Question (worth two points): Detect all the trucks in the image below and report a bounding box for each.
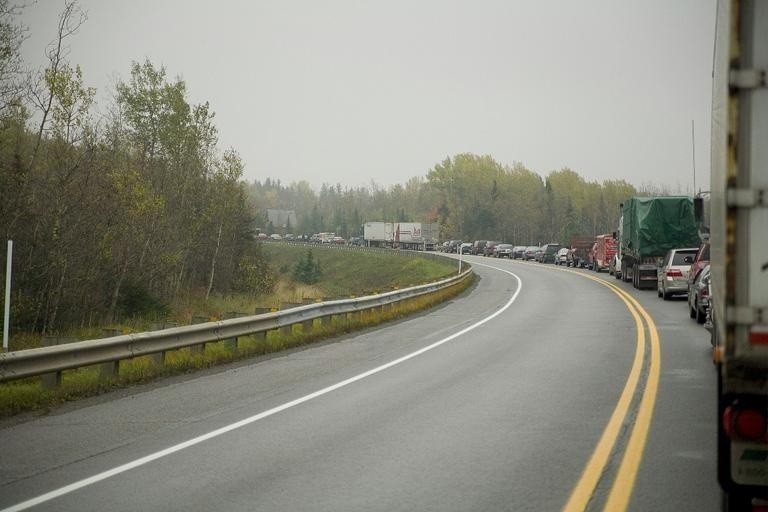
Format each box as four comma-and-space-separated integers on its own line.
693, 0, 768, 510
567, 197, 700, 323
363, 222, 440, 250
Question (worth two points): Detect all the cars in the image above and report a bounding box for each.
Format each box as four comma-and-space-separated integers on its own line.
658, 240, 712, 324
258, 232, 368, 245
442, 240, 567, 264
